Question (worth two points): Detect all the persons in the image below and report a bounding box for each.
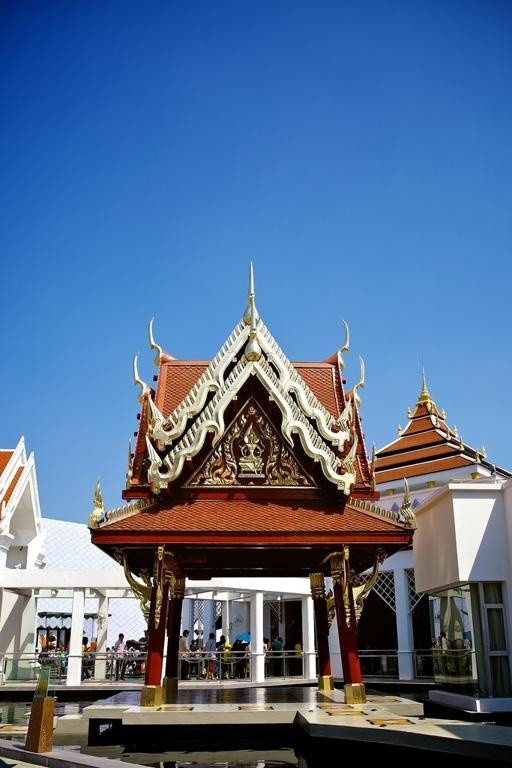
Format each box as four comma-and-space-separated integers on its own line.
81, 630, 303, 681
